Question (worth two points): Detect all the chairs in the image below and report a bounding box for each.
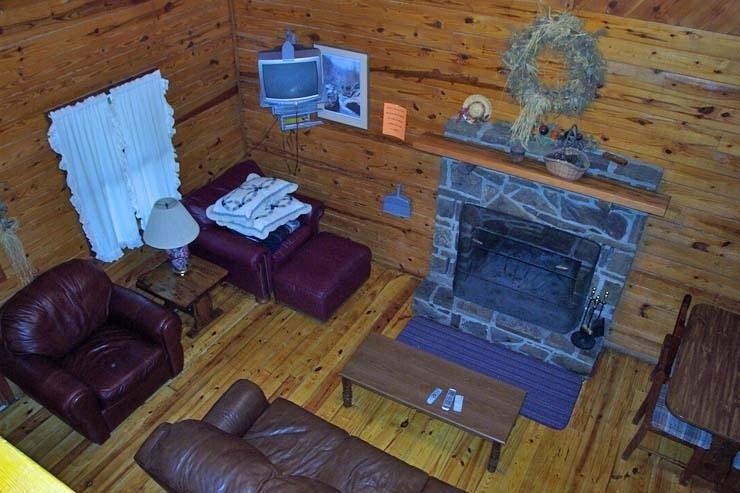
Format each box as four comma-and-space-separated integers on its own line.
674, 293, 693, 332
182, 159, 325, 303
0, 258, 185, 444
621, 336, 711, 485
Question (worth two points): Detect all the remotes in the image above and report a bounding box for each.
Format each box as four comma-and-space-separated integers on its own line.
441, 388, 456, 412
426, 388, 442, 405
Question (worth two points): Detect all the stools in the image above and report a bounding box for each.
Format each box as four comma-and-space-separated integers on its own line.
271, 230, 371, 319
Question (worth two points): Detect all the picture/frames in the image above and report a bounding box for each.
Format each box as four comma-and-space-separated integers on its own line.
313, 42, 369, 130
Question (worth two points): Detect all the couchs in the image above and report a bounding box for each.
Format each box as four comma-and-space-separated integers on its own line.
134, 381, 466, 493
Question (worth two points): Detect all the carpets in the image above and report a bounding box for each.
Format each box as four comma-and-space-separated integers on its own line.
398, 318, 583, 432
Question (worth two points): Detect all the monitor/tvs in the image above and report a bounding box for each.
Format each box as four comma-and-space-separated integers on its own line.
257, 44, 323, 104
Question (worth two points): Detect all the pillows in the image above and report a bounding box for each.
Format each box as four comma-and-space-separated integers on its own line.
207, 173, 297, 223
225, 198, 313, 240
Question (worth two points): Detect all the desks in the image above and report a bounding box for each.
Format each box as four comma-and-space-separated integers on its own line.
136, 255, 228, 336
665, 302, 739, 442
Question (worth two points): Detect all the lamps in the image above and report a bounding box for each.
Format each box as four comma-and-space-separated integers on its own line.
142, 198, 200, 274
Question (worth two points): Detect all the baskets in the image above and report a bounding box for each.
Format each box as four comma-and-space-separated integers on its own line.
544, 124, 591, 180
